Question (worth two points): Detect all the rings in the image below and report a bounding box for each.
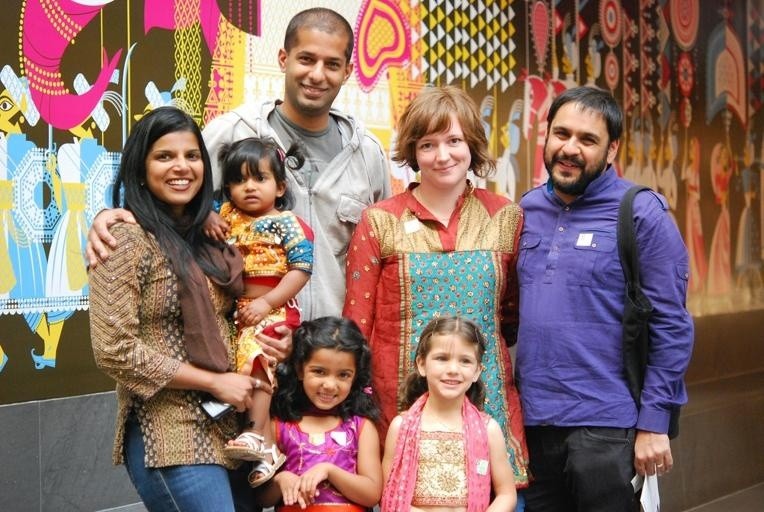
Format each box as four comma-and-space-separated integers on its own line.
253, 378, 262, 390
656, 461, 665, 471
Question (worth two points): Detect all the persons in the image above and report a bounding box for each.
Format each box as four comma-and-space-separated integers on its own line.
265, 315, 383, 512
86, 7, 395, 326
379, 315, 519, 511
86, 106, 278, 510
342, 88, 537, 511
203, 133, 315, 487
509, 86, 695, 512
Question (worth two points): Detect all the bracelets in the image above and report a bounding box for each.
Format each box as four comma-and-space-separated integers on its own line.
257, 293, 276, 313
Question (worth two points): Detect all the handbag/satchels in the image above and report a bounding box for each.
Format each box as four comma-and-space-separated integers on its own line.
623, 282, 679, 439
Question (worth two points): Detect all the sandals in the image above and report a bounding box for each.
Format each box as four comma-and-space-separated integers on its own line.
248, 444, 287, 488
225, 432, 266, 461
